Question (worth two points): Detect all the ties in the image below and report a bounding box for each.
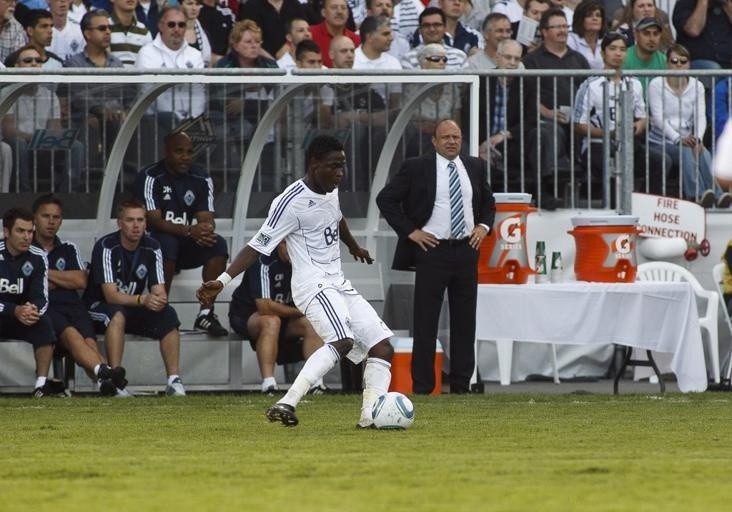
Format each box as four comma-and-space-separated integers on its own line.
447, 162, 465, 240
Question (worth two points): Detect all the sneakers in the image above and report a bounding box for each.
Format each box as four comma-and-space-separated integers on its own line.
101, 364, 128, 390
34, 378, 67, 397
307, 382, 337, 395
354, 421, 378, 430
716, 192, 731, 208
119, 389, 136, 399
100, 378, 117, 397
265, 404, 298, 427
194, 306, 228, 337
261, 381, 279, 393
165, 378, 186, 397
700, 189, 715, 208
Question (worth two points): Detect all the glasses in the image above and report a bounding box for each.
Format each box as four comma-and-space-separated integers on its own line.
421, 22, 444, 29
544, 25, 570, 30
238, 40, 263, 44
497, 51, 522, 62
85, 25, 113, 32
670, 57, 688, 64
163, 21, 186, 28
425, 55, 448, 63
16, 57, 43, 64
487, 27, 514, 35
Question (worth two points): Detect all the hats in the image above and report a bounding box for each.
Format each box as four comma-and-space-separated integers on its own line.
635, 17, 663, 33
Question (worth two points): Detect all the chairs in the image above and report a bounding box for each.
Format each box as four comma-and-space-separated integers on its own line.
630, 260, 722, 388
467, 336, 566, 389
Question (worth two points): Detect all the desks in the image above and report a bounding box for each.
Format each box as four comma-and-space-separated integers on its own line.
391, 279, 695, 397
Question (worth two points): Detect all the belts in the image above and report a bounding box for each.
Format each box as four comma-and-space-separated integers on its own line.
438, 236, 471, 245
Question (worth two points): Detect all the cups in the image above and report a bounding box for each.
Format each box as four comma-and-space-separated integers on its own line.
533, 239, 549, 284
551, 249, 567, 283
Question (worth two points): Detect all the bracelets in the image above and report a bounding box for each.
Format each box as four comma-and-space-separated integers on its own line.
135, 295, 142, 306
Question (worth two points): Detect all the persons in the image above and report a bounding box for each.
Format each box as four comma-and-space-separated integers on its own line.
412, 0, 478, 53
366, 0, 410, 61
227, 238, 344, 399
44, 0, 88, 66
196, 134, 395, 431
0, 207, 67, 399
572, 33, 672, 210
210, 20, 280, 182
134, 6, 211, 165
521, 9, 593, 211
510, 0, 553, 57
714, 117, 732, 189
134, 131, 230, 337
309, 0, 360, 67
461, 39, 536, 167
105, 0, 153, 71
375, 117, 497, 396
4, 8, 64, 70
0, 46, 84, 195
327, 35, 389, 170
349, 15, 403, 109
29, 192, 129, 398
270, 38, 334, 160
647, 44, 732, 210
566, 0, 608, 70
0, 0, 30, 65
400, 6, 468, 91
708, 77, 732, 150
63, 9, 136, 180
672, 0, 732, 89
239, 0, 309, 38
621, 16, 669, 107
275, 19, 314, 67
551, 0, 575, 32
616, 0, 672, 49
460, 12, 525, 73
81, 193, 185, 399
401, 43, 462, 136
178, 0, 212, 68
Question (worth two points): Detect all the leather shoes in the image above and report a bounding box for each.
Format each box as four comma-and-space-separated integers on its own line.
535, 193, 563, 210
451, 389, 470, 394
